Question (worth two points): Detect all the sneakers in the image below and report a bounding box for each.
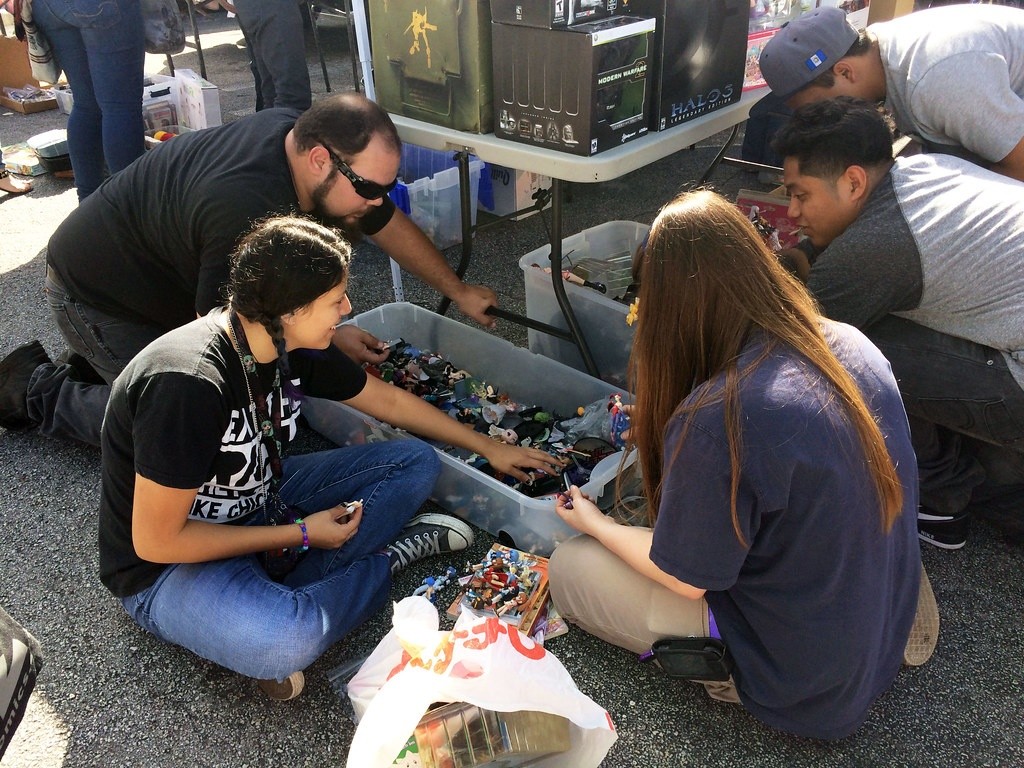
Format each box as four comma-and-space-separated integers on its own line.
256, 671, 305, 701
378, 511, 473, 575
912, 500, 968, 551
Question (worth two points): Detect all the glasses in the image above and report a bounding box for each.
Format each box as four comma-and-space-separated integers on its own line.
302, 132, 398, 200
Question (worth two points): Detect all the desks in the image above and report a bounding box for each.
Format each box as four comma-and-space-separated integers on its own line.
385, 85, 787, 380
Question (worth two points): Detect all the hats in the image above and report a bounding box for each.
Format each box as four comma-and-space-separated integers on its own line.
748, 8, 860, 119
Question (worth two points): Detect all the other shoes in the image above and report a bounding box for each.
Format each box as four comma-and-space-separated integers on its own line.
0, 340, 48, 433
897, 558, 939, 665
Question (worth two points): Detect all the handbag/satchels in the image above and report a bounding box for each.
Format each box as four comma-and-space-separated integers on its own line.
139, 0, 185, 53
346, 594, 618, 768
19, 0, 64, 84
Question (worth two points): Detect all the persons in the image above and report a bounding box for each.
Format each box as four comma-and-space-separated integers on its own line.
98, 210, 567, 702
770, 95, 1024, 551
546, 189, 940, 743
0, 149, 33, 193
0, 0, 312, 206
0, 92, 499, 450
742, 90, 792, 175
358, 339, 622, 620
759, 3, 1024, 184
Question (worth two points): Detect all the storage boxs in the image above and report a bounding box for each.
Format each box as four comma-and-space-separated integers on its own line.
300, 302, 639, 557
518, 220, 652, 391
477, 160, 551, 221
387, 140, 485, 251
0, 34, 222, 175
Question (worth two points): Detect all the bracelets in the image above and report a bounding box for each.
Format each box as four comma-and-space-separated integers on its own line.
291, 517, 308, 553
